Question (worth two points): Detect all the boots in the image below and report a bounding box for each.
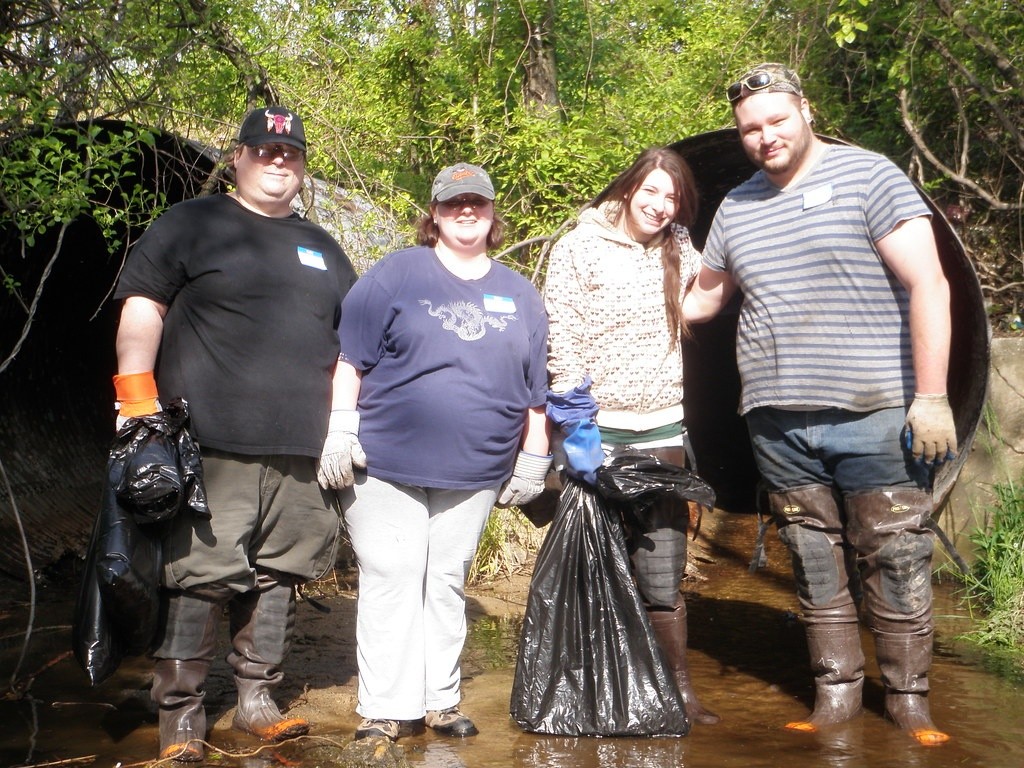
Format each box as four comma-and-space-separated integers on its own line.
767, 482, 866, 731
627, 446, 721, 726
226, 570, 310, 742
150, 582, 229, 762
845, 487, 951, 747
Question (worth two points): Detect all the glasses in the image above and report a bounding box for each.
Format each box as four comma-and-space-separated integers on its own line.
726, 73, 799, 102
253, 143, 300, 160
443, 197, 489, 211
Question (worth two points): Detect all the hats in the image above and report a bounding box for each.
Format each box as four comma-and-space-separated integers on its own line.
239, 107, 306, 151
734, 63, 802, 104
431, 162, 495, 203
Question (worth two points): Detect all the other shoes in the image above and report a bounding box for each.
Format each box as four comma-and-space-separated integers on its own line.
355, 718, 399, 742
424, 706, 478, 738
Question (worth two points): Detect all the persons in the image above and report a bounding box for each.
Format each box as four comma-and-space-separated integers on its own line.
543, 146, 738, 721
682, 63, 959, 744
316, 163, 553, 741
112, 107, 363, 762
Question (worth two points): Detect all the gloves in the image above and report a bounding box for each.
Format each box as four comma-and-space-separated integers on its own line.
112, 371, 163, 434
905, 393, 957, 466
315, 411, 368, 490
546, 377, 604, 485
498, 450, 553, 505
